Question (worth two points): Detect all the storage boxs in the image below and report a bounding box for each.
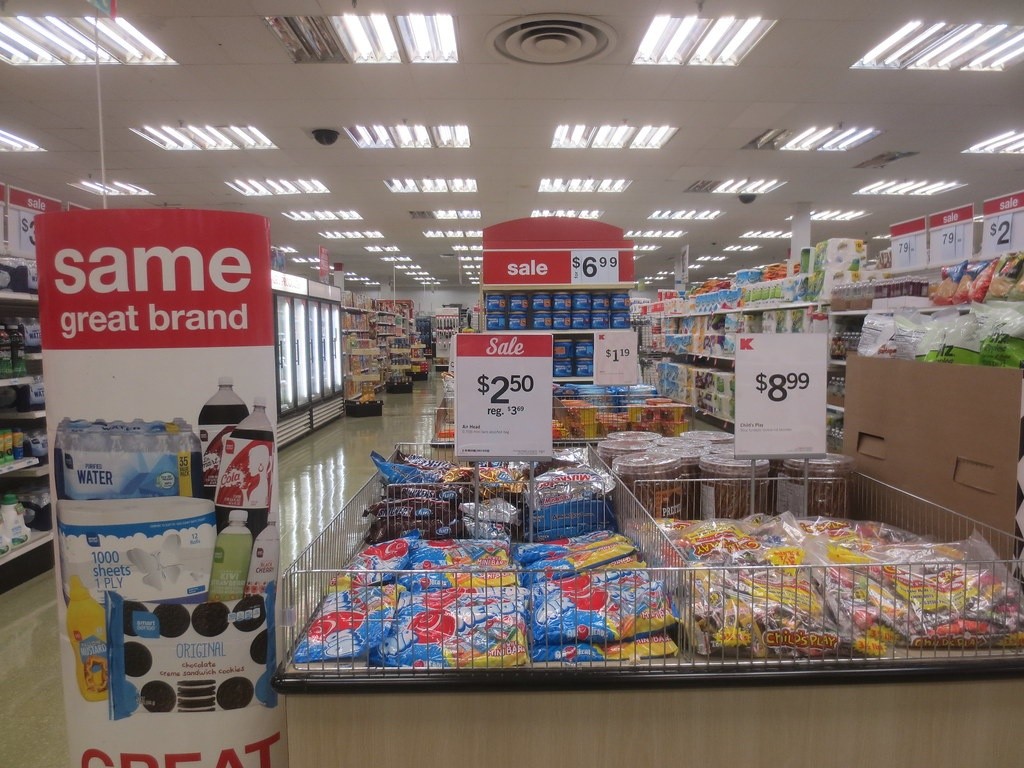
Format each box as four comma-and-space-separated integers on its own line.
406, 371, 428, 381
436, 365, 449, 371
386, 380, 413, 394
346, 402, 383, 417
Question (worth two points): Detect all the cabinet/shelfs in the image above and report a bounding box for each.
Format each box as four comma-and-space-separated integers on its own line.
628, 257, 998, 427
0, 290, 58, 593
340, 306, 425, 400
480, 283, 635, 382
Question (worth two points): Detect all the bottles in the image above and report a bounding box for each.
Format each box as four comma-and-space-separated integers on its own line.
214, 396, 275, 537
242, 512, 280, 601
2, 494, 31, 549
53, 417, 205, 499
0, 316, 41, 376
206, 510, 253, 605
199, 374, 250, 503
0, 428, 24, 463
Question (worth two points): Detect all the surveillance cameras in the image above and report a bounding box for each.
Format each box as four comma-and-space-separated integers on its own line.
738, 194, 757, 204
311, 130, 340, 146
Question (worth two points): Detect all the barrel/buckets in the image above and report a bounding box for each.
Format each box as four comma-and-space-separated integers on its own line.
598, 430, 857, 521
